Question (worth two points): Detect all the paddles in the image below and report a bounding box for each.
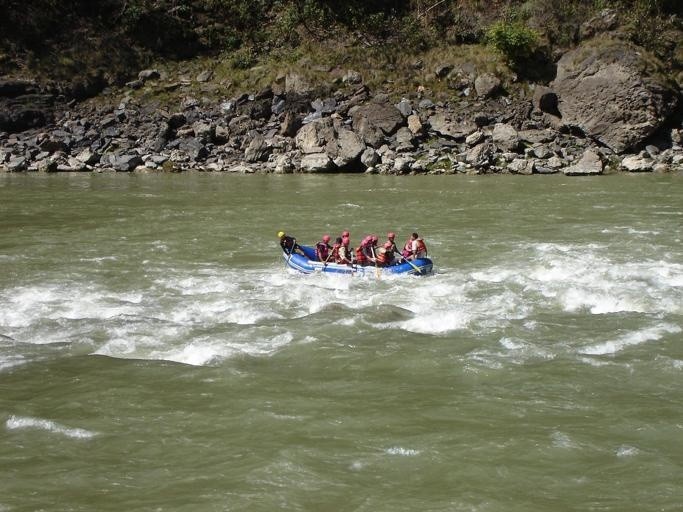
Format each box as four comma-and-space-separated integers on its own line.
394, 248, 422, 274
370, 246, 381, 278
351, 248, 358, 277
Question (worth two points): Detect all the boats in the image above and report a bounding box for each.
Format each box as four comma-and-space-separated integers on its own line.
282, 243, 434, 279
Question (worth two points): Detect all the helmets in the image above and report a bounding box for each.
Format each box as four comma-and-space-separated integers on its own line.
322, 231, 350, 245
278, 231, 285, 238
366, 233, 395, 241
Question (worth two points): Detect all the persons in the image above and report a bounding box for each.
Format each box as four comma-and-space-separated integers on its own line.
314, 231, 427, 268
277, 231, 311, 260
